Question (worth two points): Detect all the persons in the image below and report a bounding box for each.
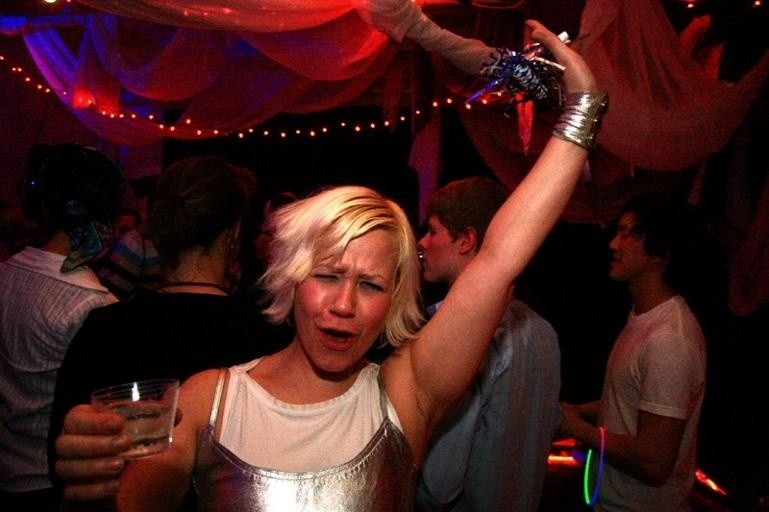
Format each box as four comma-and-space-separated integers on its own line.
54, 20, 608, 512
556, 193, 708, 512
1, 142, 297, 512
413, 175, 562, 512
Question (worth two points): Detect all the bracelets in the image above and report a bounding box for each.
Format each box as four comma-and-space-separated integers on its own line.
551, 92, 609, 152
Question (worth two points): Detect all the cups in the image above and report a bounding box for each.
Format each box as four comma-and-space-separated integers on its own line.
89, 377, 180, 461
